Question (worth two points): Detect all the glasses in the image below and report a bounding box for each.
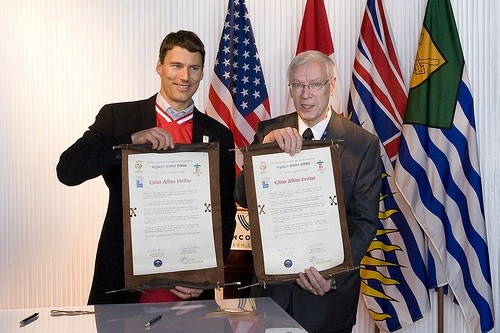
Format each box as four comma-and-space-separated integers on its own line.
287, 78, 331, 91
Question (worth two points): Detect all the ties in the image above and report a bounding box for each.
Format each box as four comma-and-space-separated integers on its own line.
302, 129, 317, 142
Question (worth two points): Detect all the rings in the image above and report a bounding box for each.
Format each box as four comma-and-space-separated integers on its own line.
308, 286, 314, 292
188, 293, 192, 299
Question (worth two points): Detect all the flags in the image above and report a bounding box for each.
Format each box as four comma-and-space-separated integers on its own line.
395, 0, 495, 333
208, 0, 271, 179
295, 0, 335, 57
347, 0, 458, 332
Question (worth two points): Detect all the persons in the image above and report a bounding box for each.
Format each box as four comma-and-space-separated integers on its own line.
233, 50, 384, 333
56, 30, 236, 305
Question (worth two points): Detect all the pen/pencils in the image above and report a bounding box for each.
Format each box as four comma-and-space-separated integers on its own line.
145, 315, 162, 326
20, 313, 39, 324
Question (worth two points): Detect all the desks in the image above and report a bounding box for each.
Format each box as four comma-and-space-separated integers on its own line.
1, 297, 308, 333
